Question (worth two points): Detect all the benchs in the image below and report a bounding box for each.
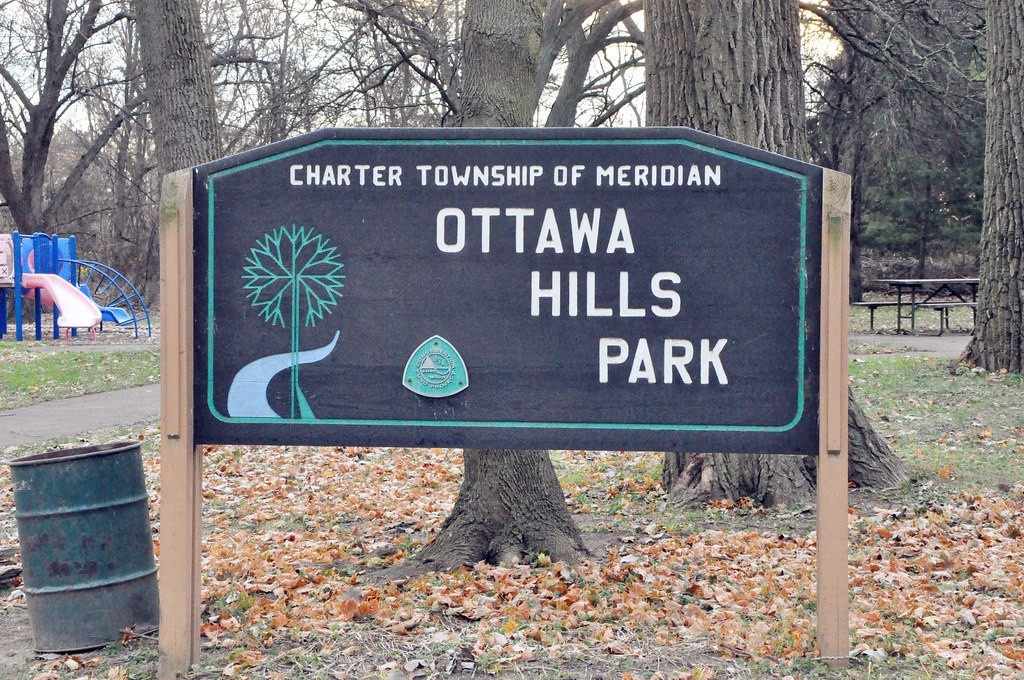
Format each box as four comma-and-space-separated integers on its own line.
851, 299, 978, 335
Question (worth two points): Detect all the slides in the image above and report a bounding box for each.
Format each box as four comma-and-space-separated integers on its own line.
20, 273, 103, 327
76, 281, 131, 324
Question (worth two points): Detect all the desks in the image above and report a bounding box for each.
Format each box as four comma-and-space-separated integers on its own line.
872, 278, 985, 336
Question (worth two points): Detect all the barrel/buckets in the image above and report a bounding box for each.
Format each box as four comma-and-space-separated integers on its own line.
7, 440, 160, 653
